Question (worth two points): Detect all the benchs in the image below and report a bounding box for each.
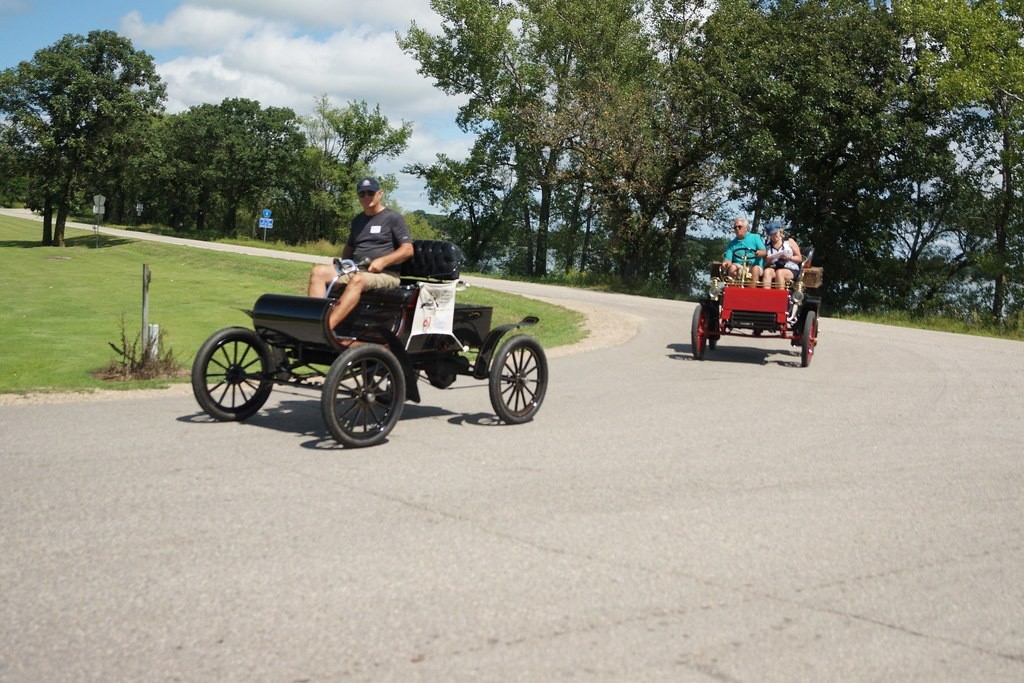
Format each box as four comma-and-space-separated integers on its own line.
325, 238, 462, 336
794, 246, 816, 281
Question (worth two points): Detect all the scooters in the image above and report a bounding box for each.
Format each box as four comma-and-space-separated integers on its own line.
690, 246, 823, 367
191, 240, 550, 448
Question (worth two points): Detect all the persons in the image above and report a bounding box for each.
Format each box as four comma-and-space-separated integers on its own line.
718, 217, 767, 289
308, 177, 415, 331
762, 222, 803, 290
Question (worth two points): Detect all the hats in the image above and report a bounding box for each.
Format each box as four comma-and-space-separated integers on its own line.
357, 178, 380, 193
765, 222, 781, 234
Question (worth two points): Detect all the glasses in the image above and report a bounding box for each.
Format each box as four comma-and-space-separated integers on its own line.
734, 225, 746, 230
357, 191, 379, 198
